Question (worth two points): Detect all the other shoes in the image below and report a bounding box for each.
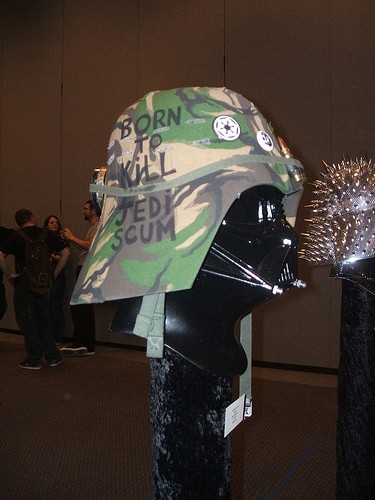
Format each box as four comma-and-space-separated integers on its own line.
18, 358, 42, 370
55, 342, 72, 351
59, 344, 95, 356
48, 352, 64, 366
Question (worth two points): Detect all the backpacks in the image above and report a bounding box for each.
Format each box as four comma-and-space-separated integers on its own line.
16, 228, 52, 295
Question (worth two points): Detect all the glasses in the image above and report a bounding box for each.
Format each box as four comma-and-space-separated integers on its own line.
48, 221, 58, 224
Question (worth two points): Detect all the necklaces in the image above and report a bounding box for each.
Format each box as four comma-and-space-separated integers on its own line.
90, 218, 99, 226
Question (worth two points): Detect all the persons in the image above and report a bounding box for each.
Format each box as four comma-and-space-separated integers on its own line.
57, 200, 103, 356
0, 199, 70, 369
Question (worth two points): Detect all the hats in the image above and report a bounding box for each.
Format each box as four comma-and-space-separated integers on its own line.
69, 83, 306, 306
